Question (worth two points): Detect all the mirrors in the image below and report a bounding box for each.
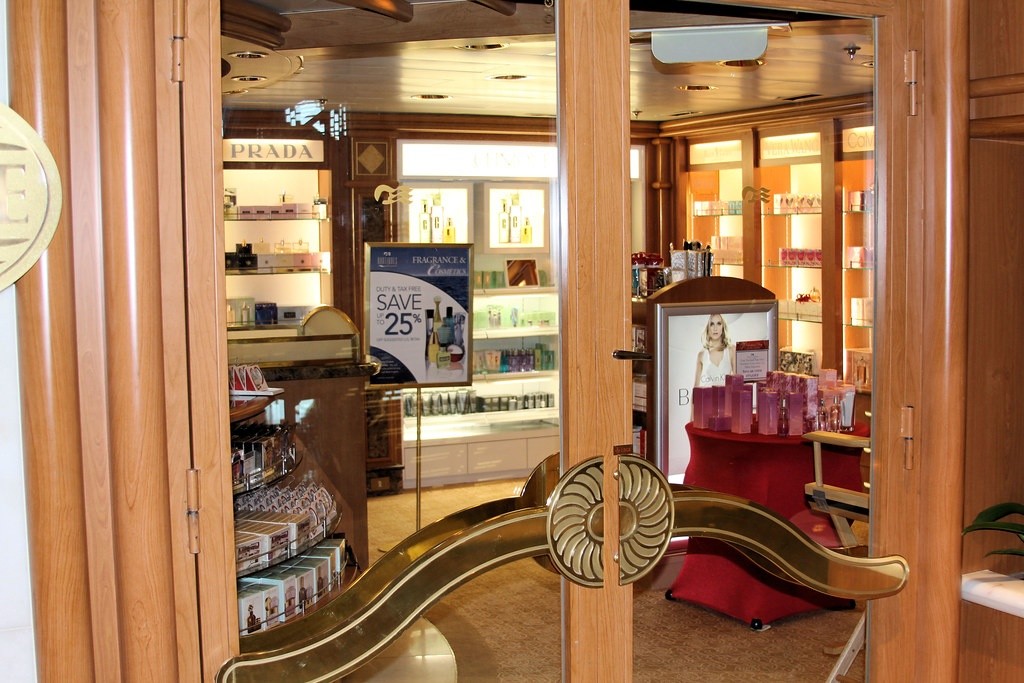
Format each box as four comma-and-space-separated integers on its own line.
504, 258, 540, 289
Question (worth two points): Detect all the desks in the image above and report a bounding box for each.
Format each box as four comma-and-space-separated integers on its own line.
666, 419, 871, 635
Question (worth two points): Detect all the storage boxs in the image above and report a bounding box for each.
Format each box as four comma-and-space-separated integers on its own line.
690, 345, 872, 435
850, 296, 874, 328
848, 190, 864, 211
227, 364, 349, 636
710, 236, 743, 265
692, 199, 742, 216
226, 242, 330, 272
475, 310, 555, 328
473, 269, 547, 290
226, 297, 313, 326
778, 247, 823, 268
847, 245, 874, 268
777, 298, 822, 317
473, 343, 556, 375
773, 193, 822, 213
238, 202, 312, 220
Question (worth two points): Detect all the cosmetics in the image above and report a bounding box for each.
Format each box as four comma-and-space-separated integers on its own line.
773, 191, 873, 386
230, 399, 345, 636
224, 193, 742, 458
692, 370, 855, 436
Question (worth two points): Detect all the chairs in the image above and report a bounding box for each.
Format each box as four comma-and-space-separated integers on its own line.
800, 425, 876, 683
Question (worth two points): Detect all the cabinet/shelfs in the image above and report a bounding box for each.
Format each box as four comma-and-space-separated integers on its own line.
226, 396, 359, 639
396, 138, 648, 491
671, 110, 878, 429
220, 162, 336, 333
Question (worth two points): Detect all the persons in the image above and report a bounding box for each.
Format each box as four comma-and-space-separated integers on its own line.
695, 313, 738, 386
